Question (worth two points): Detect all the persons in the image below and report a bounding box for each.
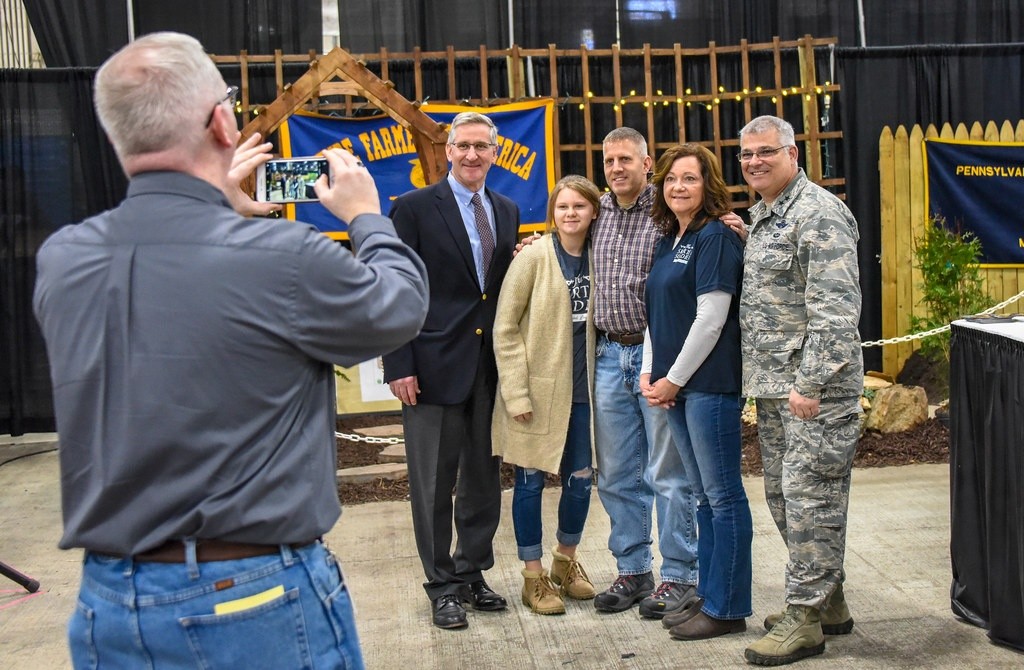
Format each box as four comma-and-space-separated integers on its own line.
512, 128, 747, 617
281, 173, 304, 199
382, 113, 522, 630
640, 144, 754, 640
491, 177, 599, 613
739, 117, 863, 665
33, 33, 428, 670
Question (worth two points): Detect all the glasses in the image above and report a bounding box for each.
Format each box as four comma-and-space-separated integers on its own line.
205, 86, 240, 129
449, 140, 496, 151
737, 144, 792, 163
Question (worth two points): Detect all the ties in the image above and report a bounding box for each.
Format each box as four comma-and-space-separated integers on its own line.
471, 193, 495, 293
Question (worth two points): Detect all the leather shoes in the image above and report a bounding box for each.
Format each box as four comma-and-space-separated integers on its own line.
431, 596, 466, 628
669, 601, 748, 641
454, 579, 507, 611
662, 592, 705, 630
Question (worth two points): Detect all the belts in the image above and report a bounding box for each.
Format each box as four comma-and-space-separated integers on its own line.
92, 533, 315, 562
598, 328, 644, 347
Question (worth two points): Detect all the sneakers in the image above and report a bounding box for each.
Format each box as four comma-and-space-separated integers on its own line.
551, 545, 595, 600
521, 566, 566, 614
594, 566, 655, 612
640, 578, 701, 618
762, 587, 854, 634
744, 600, 824, 664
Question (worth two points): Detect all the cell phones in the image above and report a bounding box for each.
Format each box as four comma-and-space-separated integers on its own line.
254, 156, 334, 204
